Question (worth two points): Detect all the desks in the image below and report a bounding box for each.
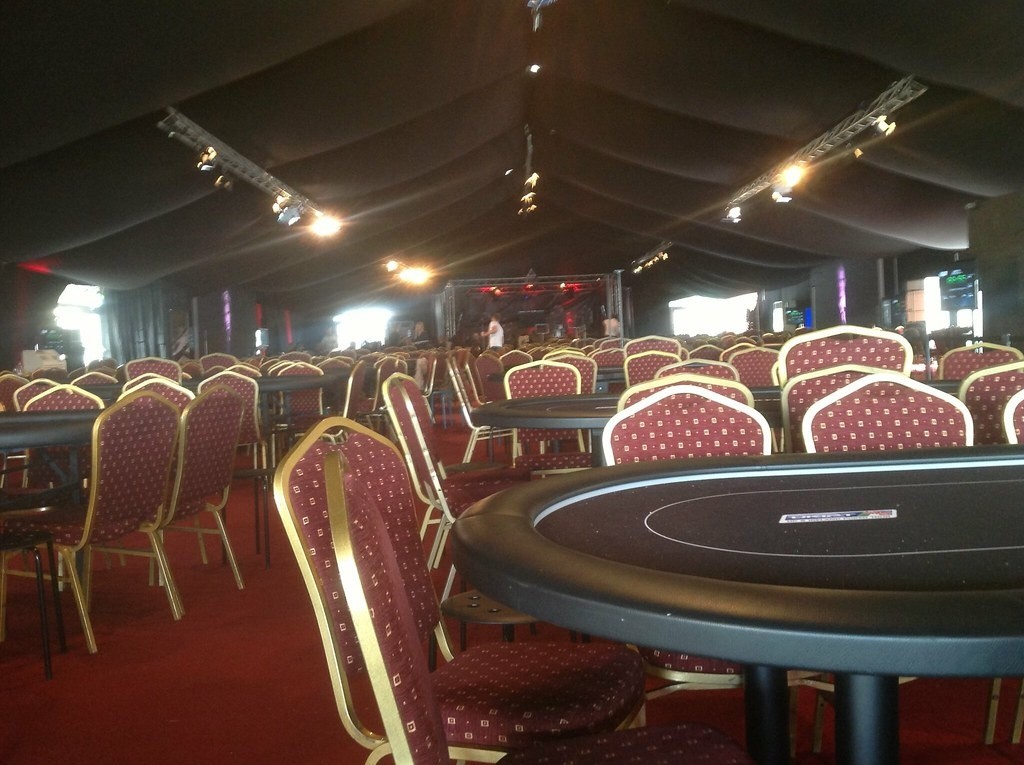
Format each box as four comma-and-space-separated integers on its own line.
485, 363, 627, 394
448, 443, 1024, 765
88, 374, 335, 437
469, 378, 962, 469
0, 408, 108, 589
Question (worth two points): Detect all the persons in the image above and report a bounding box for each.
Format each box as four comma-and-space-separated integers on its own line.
482, 313, 504, 348
602, 314, 621, 337
321, 321, 429, 352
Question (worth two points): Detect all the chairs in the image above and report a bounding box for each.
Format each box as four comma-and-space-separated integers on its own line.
0, 323, 1024, 765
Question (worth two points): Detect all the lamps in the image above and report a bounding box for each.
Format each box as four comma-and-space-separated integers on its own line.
871, 116, 892, 133
720, 202, 743, 224
270, 194, 302, 228
628, 236, 674, 277
189, 143, 232, 193
771, 169, 794, 202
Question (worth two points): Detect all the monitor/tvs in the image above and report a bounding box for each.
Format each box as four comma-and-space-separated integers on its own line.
940, 273, 979, 311
